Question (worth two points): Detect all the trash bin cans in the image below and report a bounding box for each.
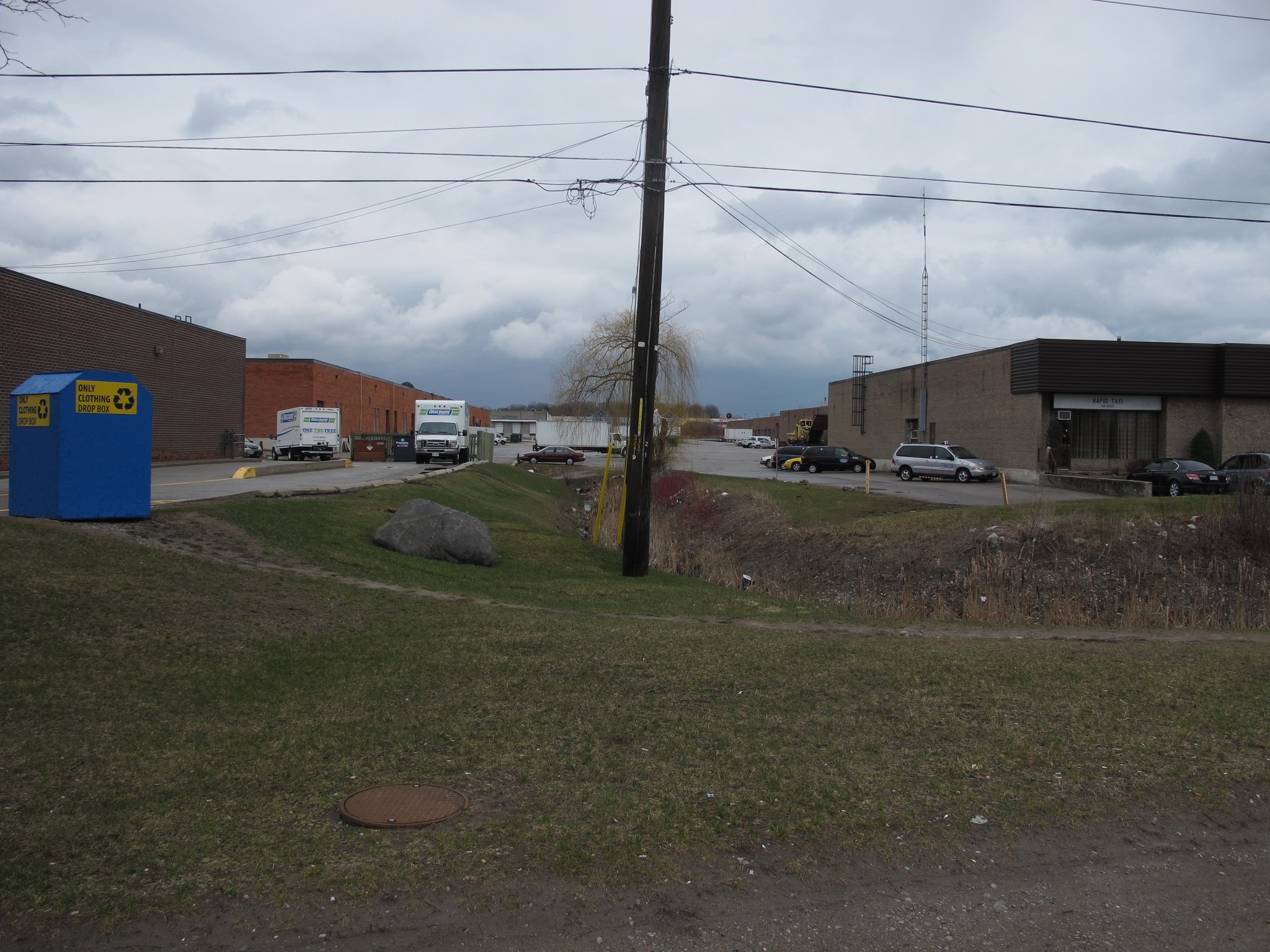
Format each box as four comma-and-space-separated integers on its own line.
393, 435, 415, 462
510, 434, 520, 442
354, 439, 386, 461
9, 369, 154, 519
512, 433, 523, 442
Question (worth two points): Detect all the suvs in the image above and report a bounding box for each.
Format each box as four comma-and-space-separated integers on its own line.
892, 441, 999, 483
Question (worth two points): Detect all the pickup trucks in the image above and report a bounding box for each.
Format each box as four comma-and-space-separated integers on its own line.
742, 437, 771, 448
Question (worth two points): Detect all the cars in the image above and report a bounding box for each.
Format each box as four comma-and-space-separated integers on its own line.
751, 440, 776, 449
522, 447, 586, 465
735, 439, 745, 446
784, 457, 802, 472
1129, 458, 1230, 497
771, 446, 808, 470
1218, 451, 1270, 496
760, 455, 779, 468
494, 437, 500, 445
801, 446, 876, 473
244, 437, 263, 459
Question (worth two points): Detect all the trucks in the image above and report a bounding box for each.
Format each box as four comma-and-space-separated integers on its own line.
494, 433, 508, 445
269, 407, 340, 461
535, 419, 626, 456
410, 399, 470, 465
